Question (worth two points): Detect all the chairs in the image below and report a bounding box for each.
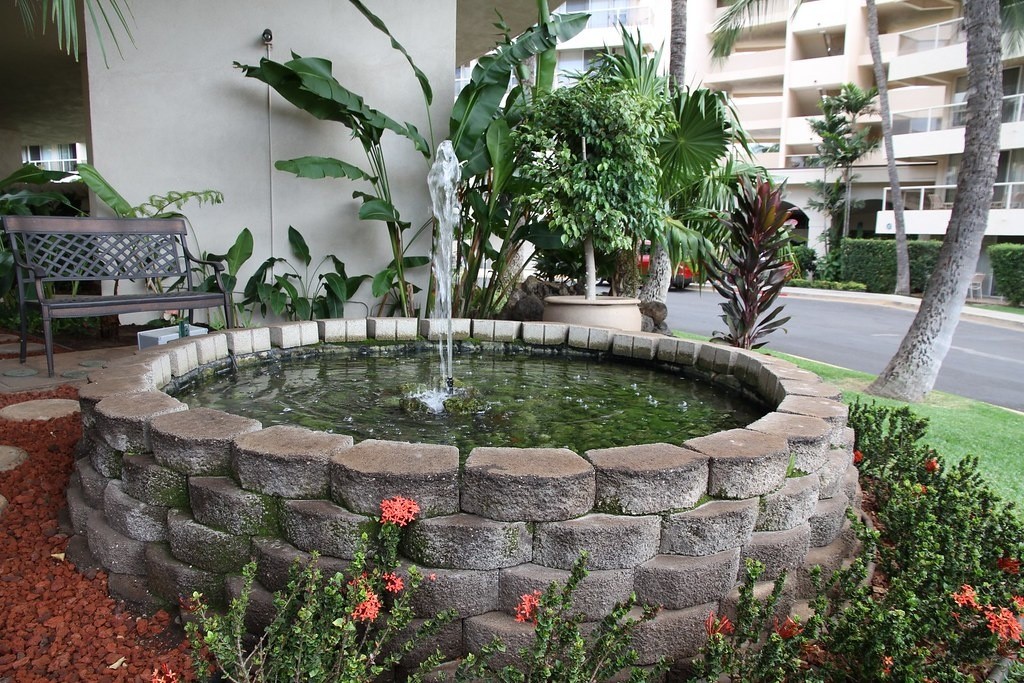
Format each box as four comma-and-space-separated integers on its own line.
969, 273, 985, 298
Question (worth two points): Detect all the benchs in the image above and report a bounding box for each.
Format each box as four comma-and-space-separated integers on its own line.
2, 215, 234, 379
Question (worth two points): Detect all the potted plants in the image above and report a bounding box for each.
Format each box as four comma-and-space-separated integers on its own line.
509, 76, 681, 332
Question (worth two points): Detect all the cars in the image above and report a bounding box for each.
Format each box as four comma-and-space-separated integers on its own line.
616, 240, 708, 290
